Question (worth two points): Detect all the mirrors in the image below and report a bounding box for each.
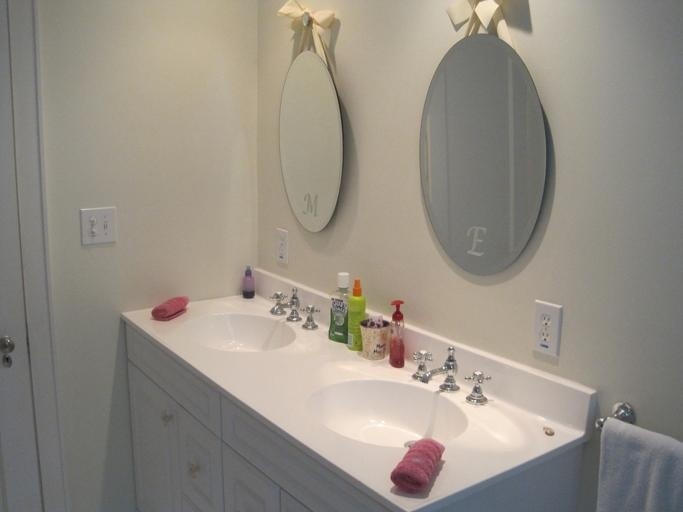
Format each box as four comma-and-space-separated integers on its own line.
419, 32, 547, 276
278, 50, 345, 234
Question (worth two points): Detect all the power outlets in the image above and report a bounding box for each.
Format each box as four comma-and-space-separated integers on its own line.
532, 299, 563, 357
275, 228, 289, 265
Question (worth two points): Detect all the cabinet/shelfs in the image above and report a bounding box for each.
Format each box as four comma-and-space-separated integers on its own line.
124, 322, 223, 511
218, 395, 394, 512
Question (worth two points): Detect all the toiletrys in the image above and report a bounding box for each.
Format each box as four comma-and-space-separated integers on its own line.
328, 272, 351, 343
389, 300, 405, 368
242, 264, 255, 298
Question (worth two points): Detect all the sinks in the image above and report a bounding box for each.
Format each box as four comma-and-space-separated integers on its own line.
309, 379, 468, 448
181, 314, 296, 352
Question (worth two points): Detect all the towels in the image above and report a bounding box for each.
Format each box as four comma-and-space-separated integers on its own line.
390, 438, 446, 492
151, 295, 189, 322
596, 417, 683, 512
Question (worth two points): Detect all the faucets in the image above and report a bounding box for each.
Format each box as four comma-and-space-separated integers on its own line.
271, 287, 302, 322
420, 346, 459, 392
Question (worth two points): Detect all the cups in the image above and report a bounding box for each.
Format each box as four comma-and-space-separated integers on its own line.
359, 319, 390, 360
